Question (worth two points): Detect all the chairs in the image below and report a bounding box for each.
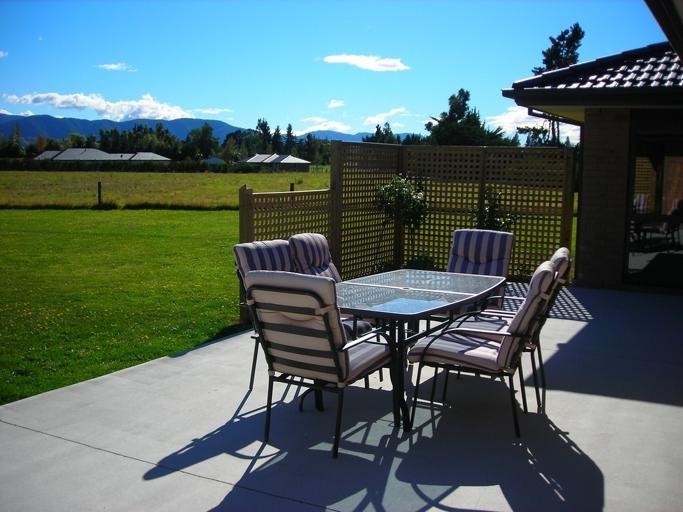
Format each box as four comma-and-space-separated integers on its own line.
244, 270, 401, 458
226, 238, 295, 430
453, 247, 574, 416
629, 193, 683, 263
289, 233, 376, 337
425, 230, 516, 337
408, 260, 558, 442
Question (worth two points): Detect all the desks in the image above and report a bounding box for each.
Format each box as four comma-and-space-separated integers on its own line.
332, 267, 507, 423
